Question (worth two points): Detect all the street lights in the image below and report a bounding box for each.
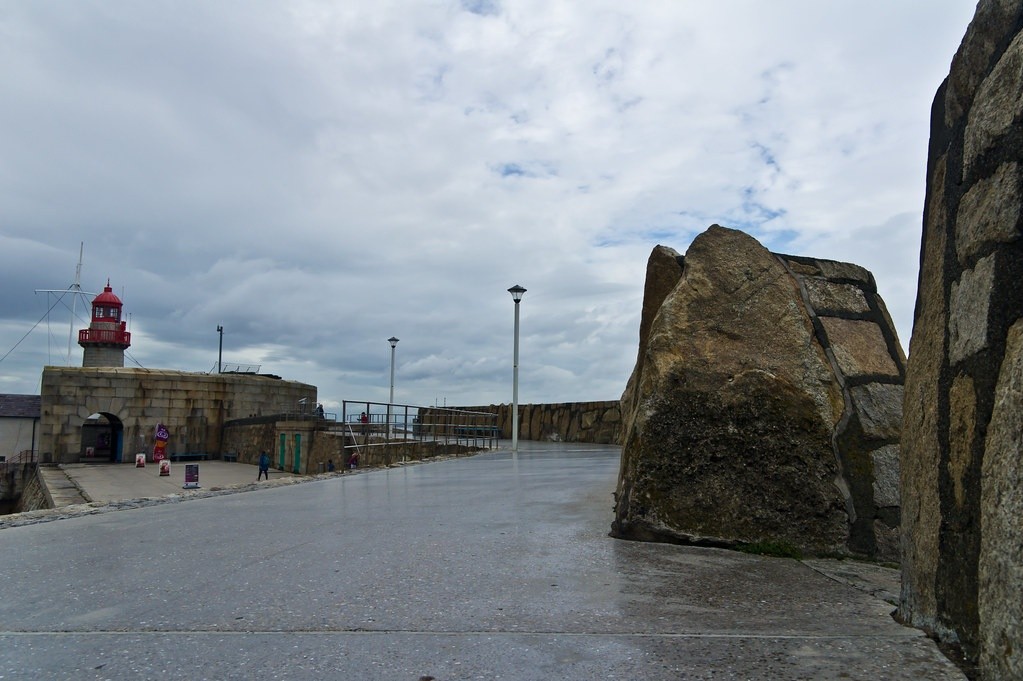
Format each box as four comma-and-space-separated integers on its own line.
508, 285, 527, 452
387, 336, 400, 439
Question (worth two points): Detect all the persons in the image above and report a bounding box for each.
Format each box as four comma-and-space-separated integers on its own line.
315, 404, 324, 419
256, 451, 270, 481
327, 458, 335, 472
356, 412, 368, 435
346, 453, 357, 469
